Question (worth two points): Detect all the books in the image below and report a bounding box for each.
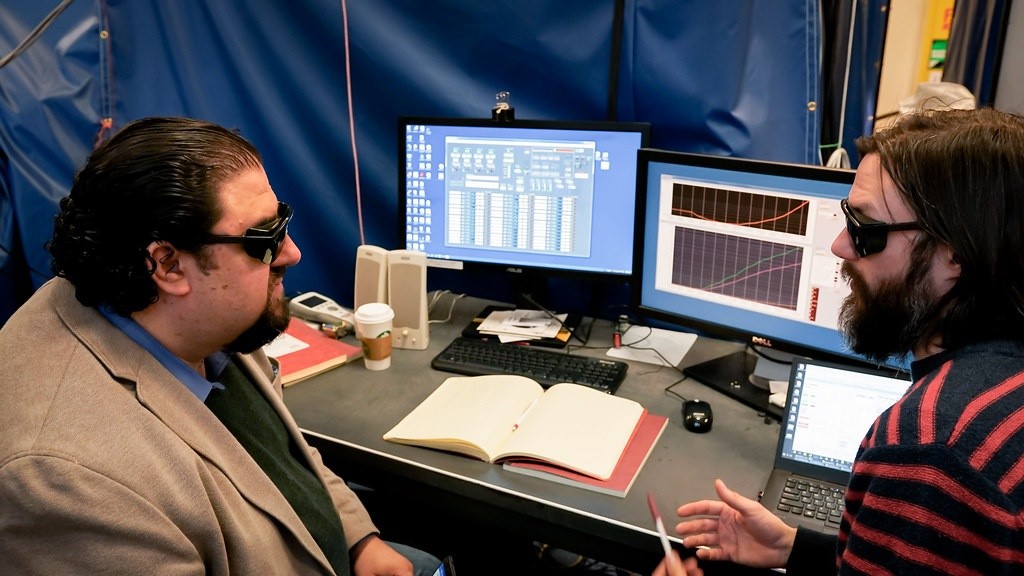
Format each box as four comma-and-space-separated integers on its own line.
380, 373, 672, 498
261, 313, 367, 388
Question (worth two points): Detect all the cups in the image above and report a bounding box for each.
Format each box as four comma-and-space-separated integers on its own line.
354, 303, 395, 372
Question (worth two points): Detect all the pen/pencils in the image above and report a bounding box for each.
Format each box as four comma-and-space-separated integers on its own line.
646, 488, 675, 563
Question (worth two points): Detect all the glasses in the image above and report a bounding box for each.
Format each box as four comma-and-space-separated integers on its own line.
199, 201, 295, 265
840, 197, 925, 258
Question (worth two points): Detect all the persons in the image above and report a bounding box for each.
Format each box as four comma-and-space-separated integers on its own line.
655, 97, 1024, 576
0, 118, 443, 576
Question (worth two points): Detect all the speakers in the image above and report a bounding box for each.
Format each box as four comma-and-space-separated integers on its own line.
354, 245, 389, 310
387, 250, 430, 351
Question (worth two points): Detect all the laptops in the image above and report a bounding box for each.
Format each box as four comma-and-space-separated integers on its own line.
760, 356, 914, 534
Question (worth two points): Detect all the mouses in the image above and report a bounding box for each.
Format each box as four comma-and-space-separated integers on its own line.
681, 398, 714, 433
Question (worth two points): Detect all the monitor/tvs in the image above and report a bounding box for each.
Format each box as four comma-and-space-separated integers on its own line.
397, 116, 652, 349
630, 146, 920, 421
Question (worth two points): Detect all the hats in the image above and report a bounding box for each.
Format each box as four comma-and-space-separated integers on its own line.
896, 81, 977, 115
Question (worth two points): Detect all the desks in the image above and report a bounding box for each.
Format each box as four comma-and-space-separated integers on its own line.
283, 290, 812, 576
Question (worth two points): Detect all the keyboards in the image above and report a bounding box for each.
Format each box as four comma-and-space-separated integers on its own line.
432, 337, 630, 395
